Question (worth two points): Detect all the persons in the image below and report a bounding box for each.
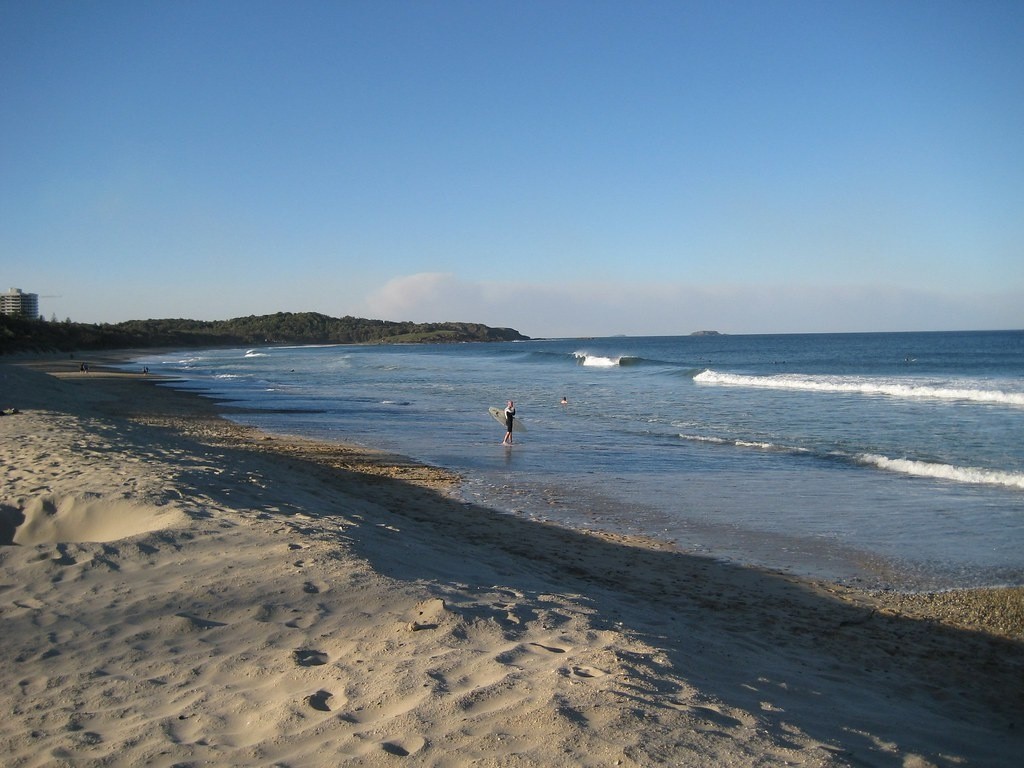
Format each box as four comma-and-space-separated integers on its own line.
561, 397, 568, 404
502, 400, 516, 446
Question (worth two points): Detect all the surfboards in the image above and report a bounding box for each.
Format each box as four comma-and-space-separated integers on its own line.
488, 406, 528, 433
